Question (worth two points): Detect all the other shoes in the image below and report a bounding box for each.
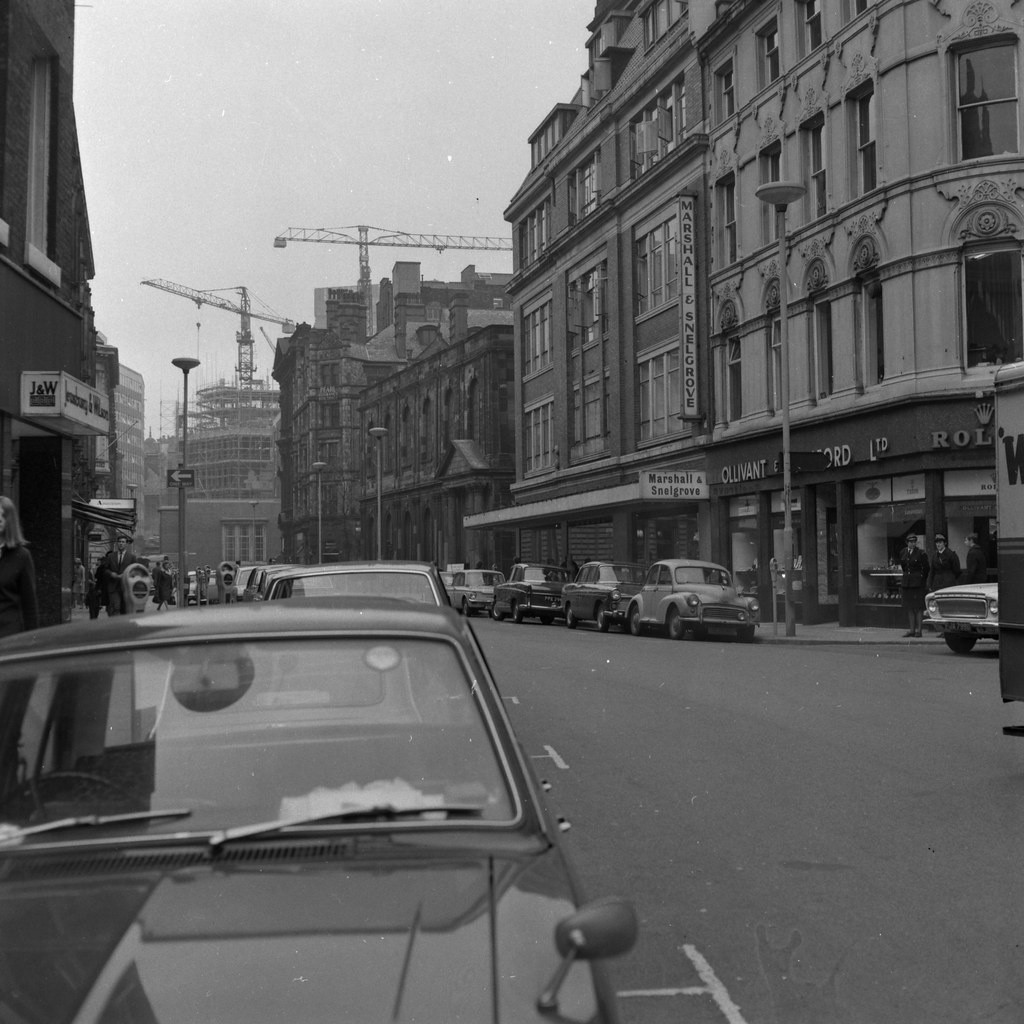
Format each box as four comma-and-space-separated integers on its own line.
916, 632, 922, 638
936, 632, 946, 638
902, 631, 914, 637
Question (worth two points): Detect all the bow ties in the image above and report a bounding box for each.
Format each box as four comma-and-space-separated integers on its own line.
937, 552, 942, 557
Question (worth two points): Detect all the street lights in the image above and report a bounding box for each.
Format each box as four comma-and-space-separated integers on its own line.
369, 426, 389, 560
126, 483, 138, 556
171, 357, 201, 610
756, 182, 810, 638
312, 460, 326, 567
249, 499, 259, 567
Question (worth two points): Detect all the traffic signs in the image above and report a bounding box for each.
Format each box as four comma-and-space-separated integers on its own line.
165, 469, 196, 488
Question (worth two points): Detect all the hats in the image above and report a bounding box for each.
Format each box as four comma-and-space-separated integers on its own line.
934, 534, 945, 542
905, 534, 917, 543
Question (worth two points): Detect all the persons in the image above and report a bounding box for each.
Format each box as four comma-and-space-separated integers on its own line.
71, 536, 279, 622
898, 534, 930, 638
925, 534, 961, 593
430, 553, 593, 593
1, 495, 40, 639
963, 533, 989, 585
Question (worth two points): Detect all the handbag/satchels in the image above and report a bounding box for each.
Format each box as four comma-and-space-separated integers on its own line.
152, 596, 161, 603
169, 596, 176, 605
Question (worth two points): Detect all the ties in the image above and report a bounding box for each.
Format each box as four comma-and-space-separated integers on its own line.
118, 552, 124, 568
908, 549, 911, 559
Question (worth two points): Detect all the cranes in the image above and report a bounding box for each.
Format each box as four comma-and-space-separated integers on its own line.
259, 325, 279, 355
141, 278, 299, 389
272, 222, 514, 340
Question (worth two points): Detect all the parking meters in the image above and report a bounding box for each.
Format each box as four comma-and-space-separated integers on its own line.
215, 560, 238, 605
769, 557, 779, 638
121, 564, 153, 617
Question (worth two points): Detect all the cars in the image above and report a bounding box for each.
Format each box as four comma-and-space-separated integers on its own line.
187, 559, 454, 609
561, 560, 653, 633
491, 563, 574, 625
437, 569, 456, 588
922, 583, 998, 655
445, 569, 507, 617
0, 593, 641, 1023
624, 559, 761, 643
